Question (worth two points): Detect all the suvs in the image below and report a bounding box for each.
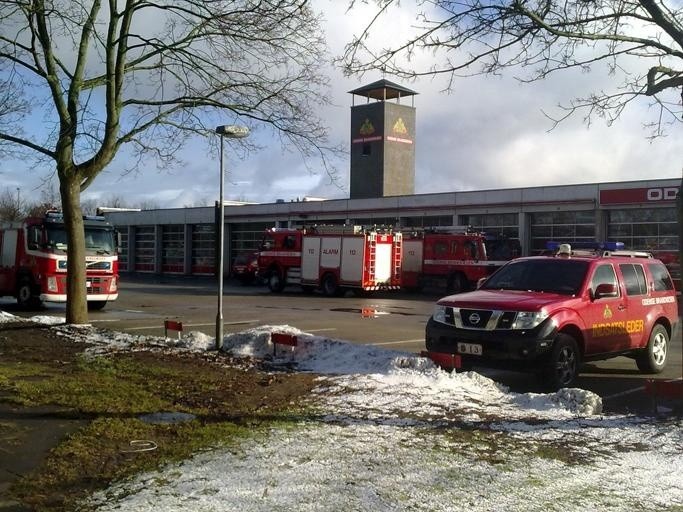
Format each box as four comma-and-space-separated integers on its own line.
230, 251, 269, 287
416, 240, 677, 389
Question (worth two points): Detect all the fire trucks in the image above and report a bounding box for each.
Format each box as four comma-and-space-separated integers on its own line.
398, 225, 522, 293
239, 220, 404, 297
0, 206, 120, 314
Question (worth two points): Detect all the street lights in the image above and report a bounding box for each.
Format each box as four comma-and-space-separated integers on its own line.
214, 122, 251, 351
